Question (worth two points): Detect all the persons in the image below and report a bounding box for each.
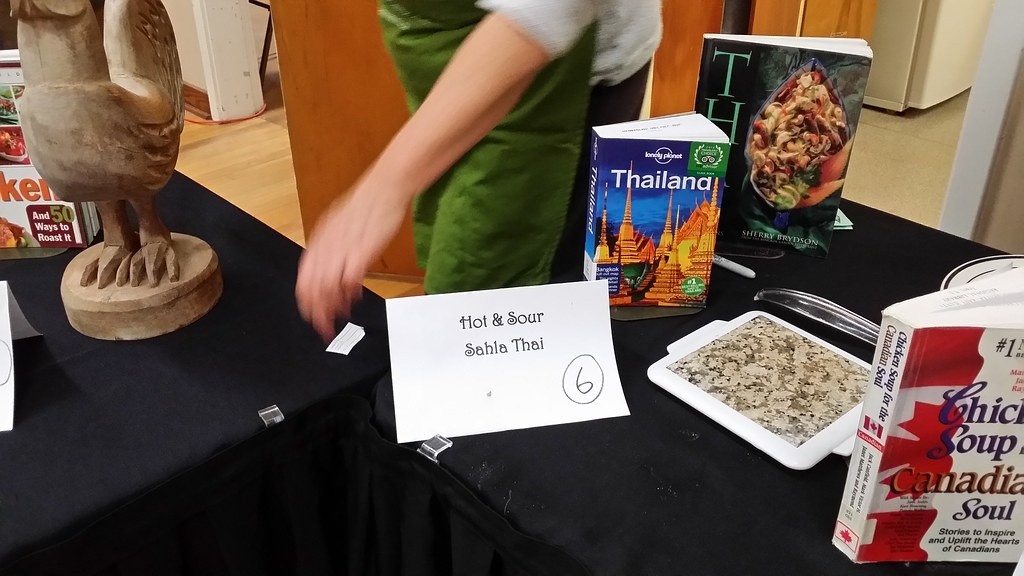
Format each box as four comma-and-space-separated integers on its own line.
293, 0, 664, 343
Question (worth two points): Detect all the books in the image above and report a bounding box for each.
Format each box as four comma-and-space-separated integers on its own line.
582, 111, 731, 309
0, 49, 100, 249
694, 33, 873, 258
831, 265, 1024, 562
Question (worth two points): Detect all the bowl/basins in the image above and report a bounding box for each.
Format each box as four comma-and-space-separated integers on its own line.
0, 149, 27, 162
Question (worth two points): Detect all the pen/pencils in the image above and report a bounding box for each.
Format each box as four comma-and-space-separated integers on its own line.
713, 254, 757, 279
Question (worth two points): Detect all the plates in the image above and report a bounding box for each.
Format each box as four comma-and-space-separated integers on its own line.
940, 255, 1024, 291
13, 87, 23, 95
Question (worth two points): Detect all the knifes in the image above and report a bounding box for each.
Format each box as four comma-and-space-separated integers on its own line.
714, 242, 785, 258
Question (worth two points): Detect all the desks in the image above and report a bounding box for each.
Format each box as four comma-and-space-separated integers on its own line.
0, 171, 401, 576
373, 194, 1022, 575
